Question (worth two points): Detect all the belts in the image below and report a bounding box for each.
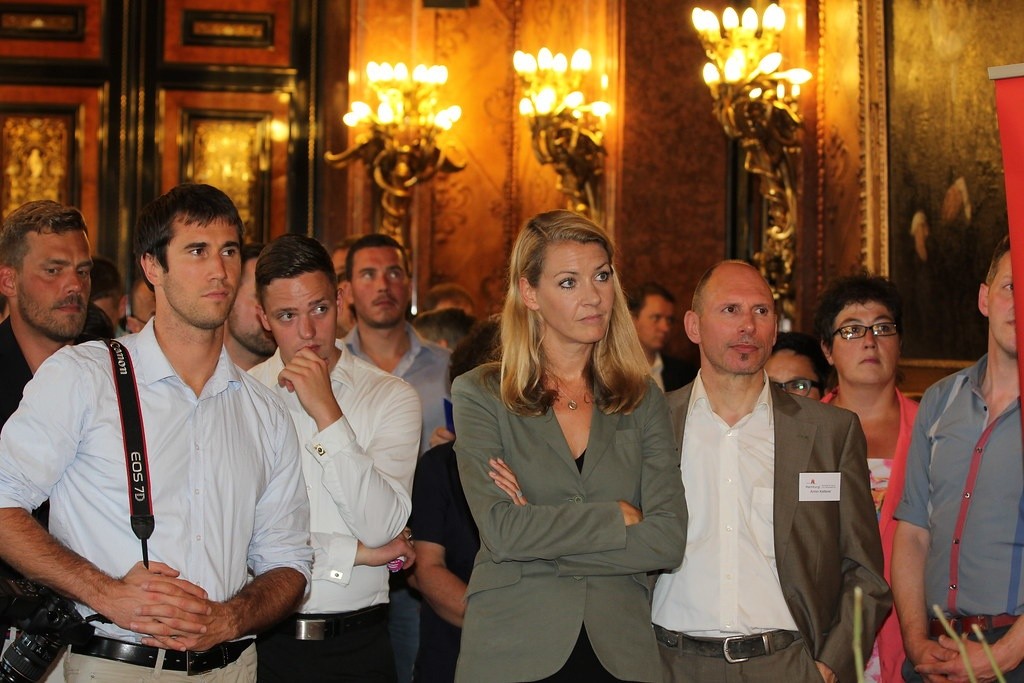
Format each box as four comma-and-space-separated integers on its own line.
274, 603, 386, 643
70, 633, 254, 677
649, 622, 802, 664
928, 613, 1021, 638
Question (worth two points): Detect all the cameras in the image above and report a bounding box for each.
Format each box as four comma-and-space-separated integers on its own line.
0, 569, 95, 683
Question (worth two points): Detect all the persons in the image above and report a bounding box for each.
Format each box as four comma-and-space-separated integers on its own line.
329, 245, 508, 456
224, 243, 279, 372
0, 198, 93, 683
765, 332, 832, 402
74, 255, 156, 346
628, 281, 698, 392
0, 184, 315, 683
814, 275, 920, 683
451, 209, 689, 683
404, 312, 505, 683
888, 232, 1024, 683
342, 234, 455, 683
245, 233, 423, 683
651, 260, 892, 683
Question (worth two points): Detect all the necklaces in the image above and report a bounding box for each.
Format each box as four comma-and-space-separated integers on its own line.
547, 373, 584, 410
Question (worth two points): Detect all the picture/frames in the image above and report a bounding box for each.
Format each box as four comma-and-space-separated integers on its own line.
856, 0, 1024, 369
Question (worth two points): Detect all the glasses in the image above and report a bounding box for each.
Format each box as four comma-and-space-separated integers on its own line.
771, 378, 824, 397
831, 321, 900, 340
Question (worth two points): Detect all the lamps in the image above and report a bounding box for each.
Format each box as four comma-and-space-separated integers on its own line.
513, 47, 613, 226
693, 2, 812, 318
324, 61, 469, 275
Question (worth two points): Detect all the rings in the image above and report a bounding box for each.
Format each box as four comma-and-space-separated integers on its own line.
408, 534, 413, 540
515, 490, 522, 498
